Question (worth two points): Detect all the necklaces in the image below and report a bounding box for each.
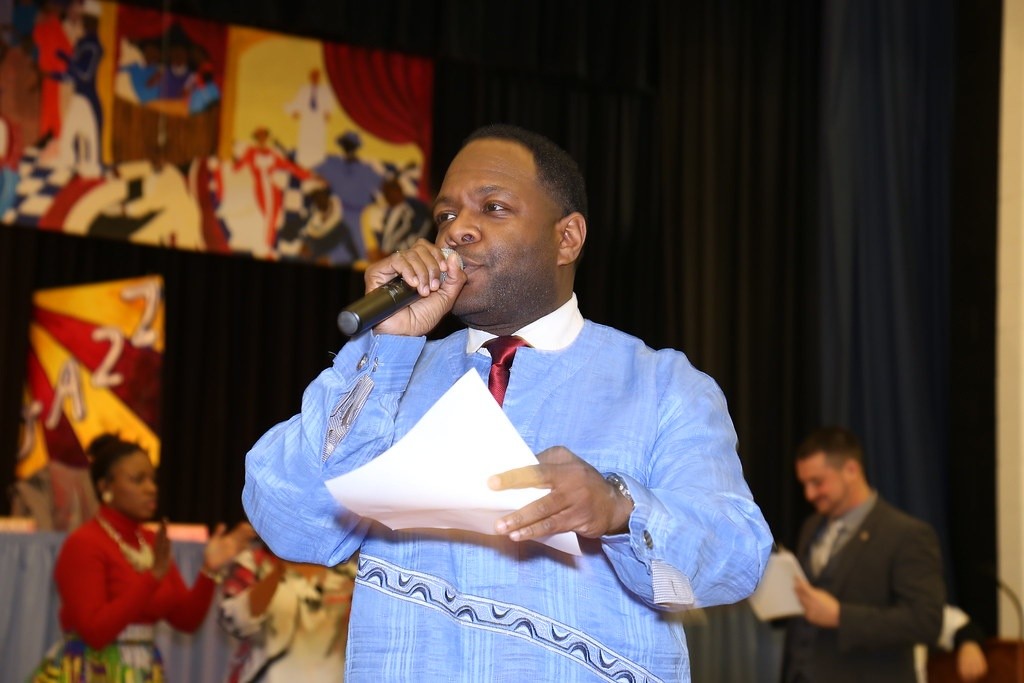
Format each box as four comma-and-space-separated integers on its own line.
96, 505, 156, 571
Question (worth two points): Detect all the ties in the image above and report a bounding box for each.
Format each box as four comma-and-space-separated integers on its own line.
810, 521, 844, 580
481, 336, 535, 407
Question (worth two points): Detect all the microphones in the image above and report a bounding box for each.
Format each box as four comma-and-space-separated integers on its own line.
338, 249, 463, 337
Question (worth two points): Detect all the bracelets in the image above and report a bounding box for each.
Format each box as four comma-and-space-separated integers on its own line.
605, 473, 634, 511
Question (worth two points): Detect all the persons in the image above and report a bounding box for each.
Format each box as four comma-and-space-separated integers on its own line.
744, 423, 950, 683
240, 128, 774, 683
54, 430, 252, 683
218, 532, 360, 683
